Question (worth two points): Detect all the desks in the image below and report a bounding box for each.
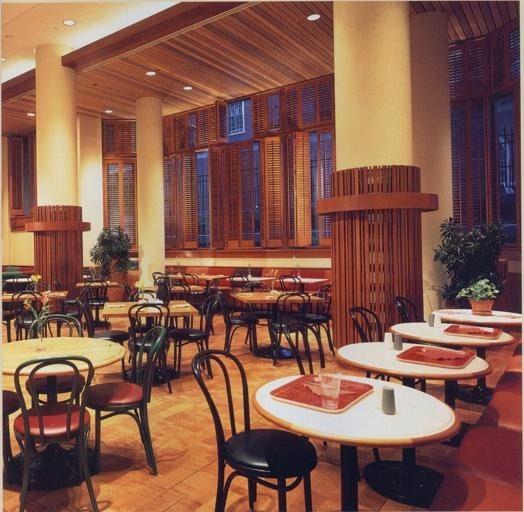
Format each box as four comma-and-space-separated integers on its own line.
335, 308, 524, 379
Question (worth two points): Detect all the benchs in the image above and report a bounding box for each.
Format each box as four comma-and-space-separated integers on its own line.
128, 266, 334, 318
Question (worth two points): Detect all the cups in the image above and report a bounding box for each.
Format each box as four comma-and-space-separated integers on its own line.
320, 368, 341, 408
433, 311, 442, 328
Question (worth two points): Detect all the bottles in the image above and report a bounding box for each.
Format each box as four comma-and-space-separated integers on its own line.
384, 333, 392, 349
382, 387, 396, 415
393, 333, 403, 350
428, 314, 435, 328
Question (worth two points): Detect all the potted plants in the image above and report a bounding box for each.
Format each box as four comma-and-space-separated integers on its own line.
88, 224, 134, 302
456, 279, 500, 316
